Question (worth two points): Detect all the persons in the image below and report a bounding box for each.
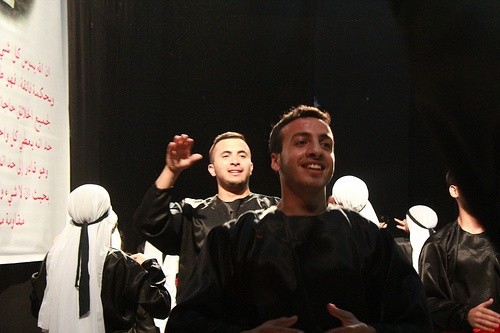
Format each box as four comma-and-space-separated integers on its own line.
417, 168, 500, 332
134, 130, 280, 305
30, 184, 171, 333
163, 105, 434, 333
331, 175, 396, 247
394, 205, 438, 277
